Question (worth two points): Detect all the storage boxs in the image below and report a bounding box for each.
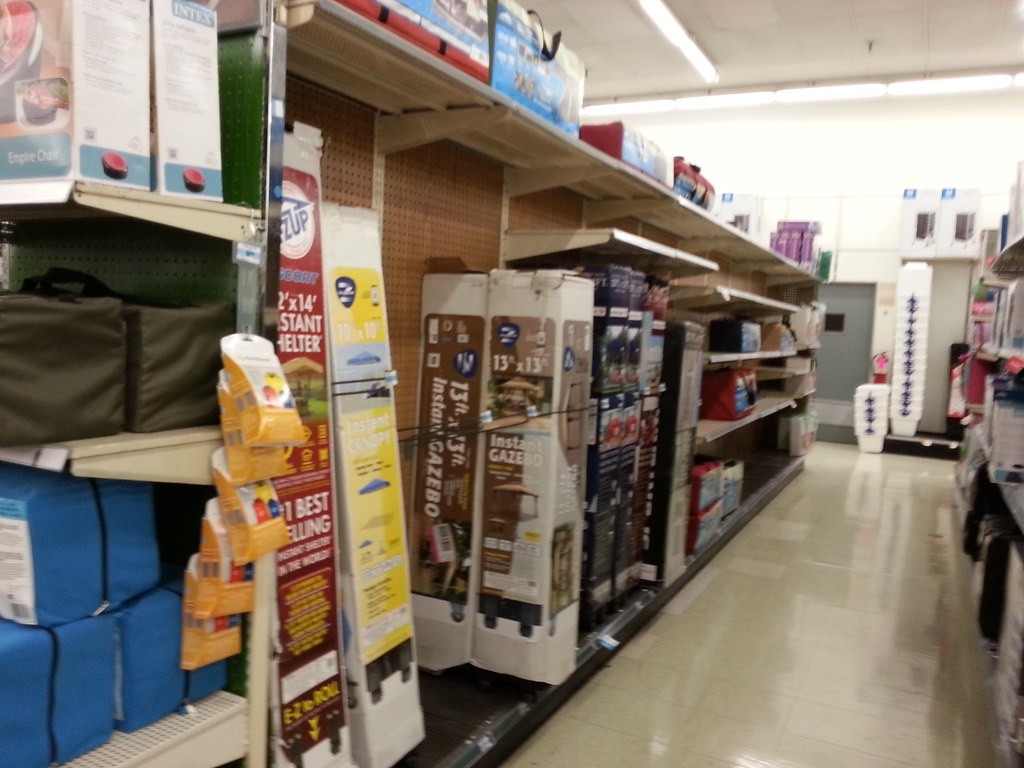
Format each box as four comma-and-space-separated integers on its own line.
0, 0, 224, 203
344, 0, 820, 624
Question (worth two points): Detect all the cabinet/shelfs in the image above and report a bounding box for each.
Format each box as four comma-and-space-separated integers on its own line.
0, 173, 267, 768
282, 0, 831, 767
961, 236, 1023, 543
